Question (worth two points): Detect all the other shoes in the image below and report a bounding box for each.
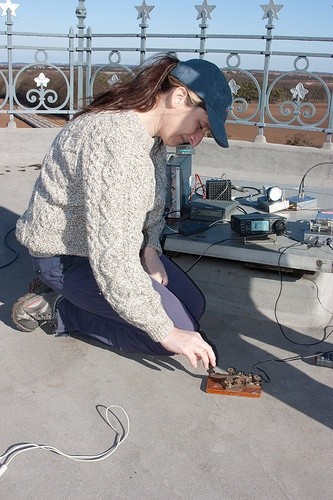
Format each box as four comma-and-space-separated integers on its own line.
28, 275, 52, 294
11, 290, 63, 332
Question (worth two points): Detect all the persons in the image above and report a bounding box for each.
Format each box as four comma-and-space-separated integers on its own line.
11, 55, 232, 370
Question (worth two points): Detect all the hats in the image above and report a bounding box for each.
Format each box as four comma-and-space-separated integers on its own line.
170, 59, 233, 149
